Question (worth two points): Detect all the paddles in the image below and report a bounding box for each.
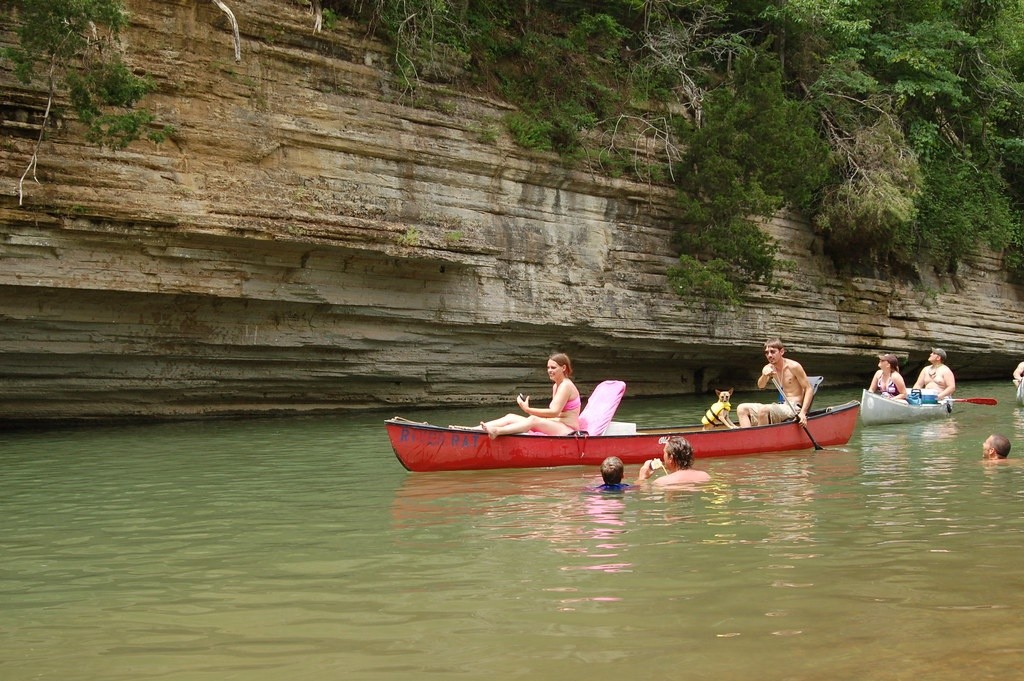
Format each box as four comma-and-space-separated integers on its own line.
520, 393, 526, 402
943, 397, 998, 406
769, 375, 825, 450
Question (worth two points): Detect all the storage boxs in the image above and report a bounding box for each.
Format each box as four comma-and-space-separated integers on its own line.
907, 388, 939, 404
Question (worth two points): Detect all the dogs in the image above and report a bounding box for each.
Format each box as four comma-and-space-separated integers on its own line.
702, 386, 742, 431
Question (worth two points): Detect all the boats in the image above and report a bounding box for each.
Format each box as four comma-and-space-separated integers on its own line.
382, 400, 862, 471
860, 388, 954, 426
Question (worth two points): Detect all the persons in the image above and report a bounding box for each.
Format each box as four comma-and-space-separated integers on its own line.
639, 436, 711, 482
1012, 361, 1024, 381
867, 354, 909, 404
736, 338, 813, 428
597, 456, 629, 490
913, 347, 956, 400
982, 434, 1011, 459
448, 354, 581, 439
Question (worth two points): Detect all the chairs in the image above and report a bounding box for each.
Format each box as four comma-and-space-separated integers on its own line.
784, 377, 824, 422
528, 380, 626, 436
